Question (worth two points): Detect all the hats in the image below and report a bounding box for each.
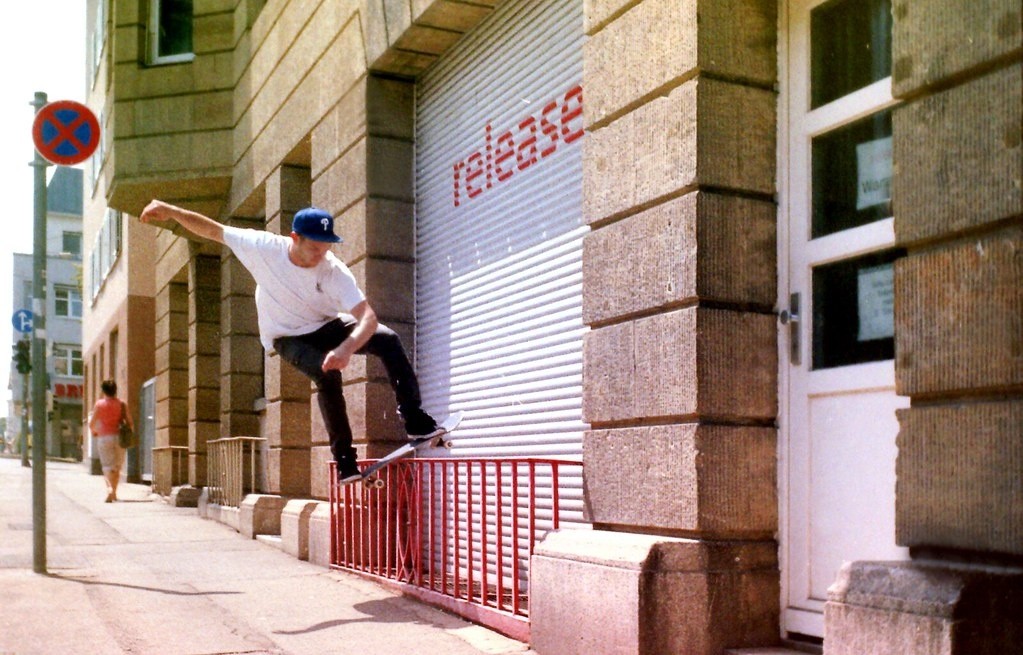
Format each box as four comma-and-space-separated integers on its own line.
291, 207, 344, 243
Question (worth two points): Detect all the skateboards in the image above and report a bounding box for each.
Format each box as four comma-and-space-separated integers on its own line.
330, 409, 470, 490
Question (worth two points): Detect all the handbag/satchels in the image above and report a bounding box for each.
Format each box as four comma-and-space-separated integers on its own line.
118, 402, 133, 449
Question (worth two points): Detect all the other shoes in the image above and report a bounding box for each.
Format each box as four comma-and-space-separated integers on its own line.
113, 494, 117, 502
105, 493, 113, 503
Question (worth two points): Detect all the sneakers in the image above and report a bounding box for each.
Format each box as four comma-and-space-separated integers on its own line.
336, 454, 363, 483
404, 413, 447, 439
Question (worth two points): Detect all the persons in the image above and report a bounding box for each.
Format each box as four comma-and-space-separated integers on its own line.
140, 199, 446, 483
89, 379, 135, 504
